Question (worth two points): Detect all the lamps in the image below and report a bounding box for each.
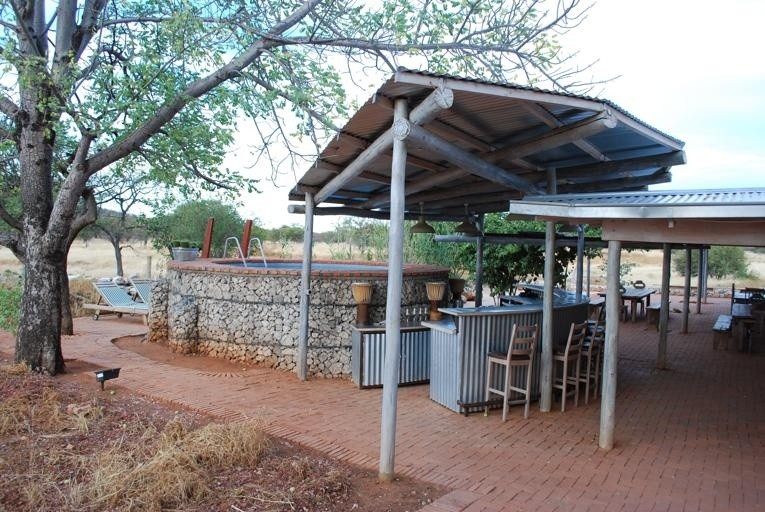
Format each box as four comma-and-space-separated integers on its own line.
558, 224, 582, 232
454, 203, 479, 233
462, 211, 486, 237
409, 202, 436, 234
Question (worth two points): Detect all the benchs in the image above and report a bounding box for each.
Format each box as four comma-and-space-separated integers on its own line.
645, 300, 671, 332
712, 314, 732, 351
589, 298, 606, 321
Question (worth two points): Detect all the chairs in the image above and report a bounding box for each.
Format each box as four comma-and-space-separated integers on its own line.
745, 310, 764, 355
484, 322, 539, 422
539, 320, 588, 412
83, 280, 150, 325
572, 302, 606, 404
128, 279, 151, 316
631, 280, 646, 314
604, 292, 628, 323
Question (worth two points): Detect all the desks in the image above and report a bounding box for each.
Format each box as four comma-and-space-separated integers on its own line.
597, 287, 657, 322
734, 292, 758, 305
732, 303, 765, 352
